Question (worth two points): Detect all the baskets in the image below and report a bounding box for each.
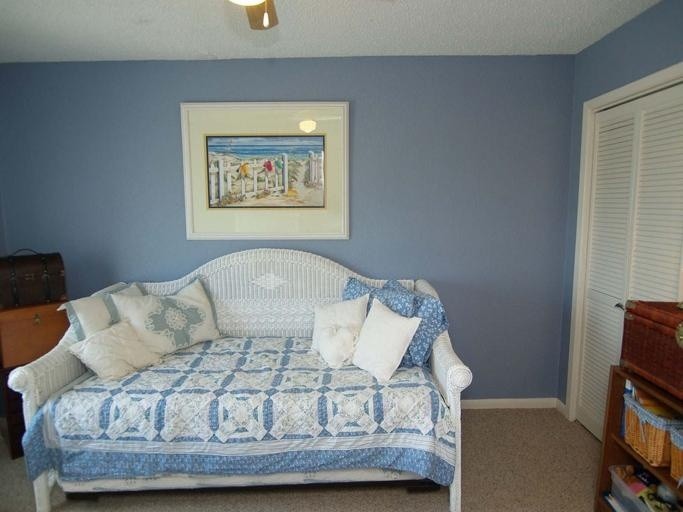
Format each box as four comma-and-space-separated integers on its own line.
622, 393, 682, 485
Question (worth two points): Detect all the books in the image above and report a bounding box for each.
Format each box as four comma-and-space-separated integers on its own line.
617, 378, 676, 439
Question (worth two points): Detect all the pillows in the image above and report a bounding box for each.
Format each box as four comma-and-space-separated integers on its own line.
56, 275, 220, 383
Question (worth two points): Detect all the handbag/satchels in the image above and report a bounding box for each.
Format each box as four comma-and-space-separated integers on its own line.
0, 247, 69, 312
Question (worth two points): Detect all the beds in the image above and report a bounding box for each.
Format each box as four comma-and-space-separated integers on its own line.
5, 246, 474, 510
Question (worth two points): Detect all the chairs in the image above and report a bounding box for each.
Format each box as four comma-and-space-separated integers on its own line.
311, 275, 447, 385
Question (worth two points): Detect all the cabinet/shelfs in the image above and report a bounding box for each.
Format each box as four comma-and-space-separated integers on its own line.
593, 364, 683, 512
569, 61, 683, 443
0, 298, 71, 459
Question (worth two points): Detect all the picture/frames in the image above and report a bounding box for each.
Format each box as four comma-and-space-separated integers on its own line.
180, 100, 350, 241
